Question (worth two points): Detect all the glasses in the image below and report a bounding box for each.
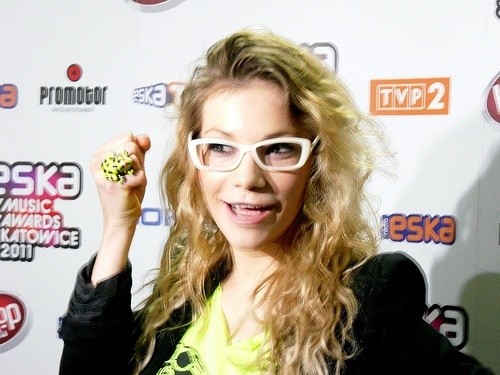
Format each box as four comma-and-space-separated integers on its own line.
187, 129, 321, 172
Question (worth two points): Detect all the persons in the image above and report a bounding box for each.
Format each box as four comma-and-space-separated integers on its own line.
57, 27, 498, 375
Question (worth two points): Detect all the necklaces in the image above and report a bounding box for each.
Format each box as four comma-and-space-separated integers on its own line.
218, 306, 257, 346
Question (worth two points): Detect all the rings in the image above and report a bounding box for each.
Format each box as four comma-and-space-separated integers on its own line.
99, 149, 135, 185
